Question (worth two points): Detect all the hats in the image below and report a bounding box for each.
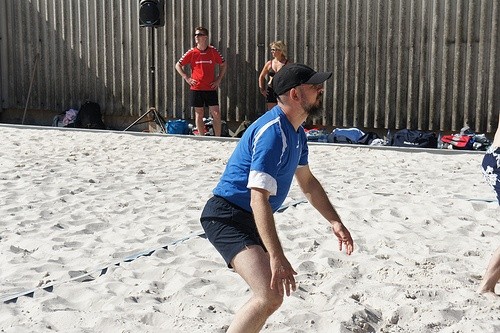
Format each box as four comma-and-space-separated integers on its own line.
271, 63, 333, 97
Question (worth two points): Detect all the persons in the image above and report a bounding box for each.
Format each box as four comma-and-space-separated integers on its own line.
200, 63, 354, 333
259, 40, 291, 112
176, 27, 227, 137
476, 124, 500, 300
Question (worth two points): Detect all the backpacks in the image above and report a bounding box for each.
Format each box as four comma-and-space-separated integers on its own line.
73, 100, 106, 130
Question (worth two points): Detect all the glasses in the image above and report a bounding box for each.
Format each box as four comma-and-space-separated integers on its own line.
194, 33, 206, 36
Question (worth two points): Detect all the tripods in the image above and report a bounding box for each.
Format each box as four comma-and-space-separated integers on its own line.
123, 27, 167, 134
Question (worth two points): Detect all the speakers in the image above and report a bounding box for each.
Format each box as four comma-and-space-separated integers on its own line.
139, 0, 165, 28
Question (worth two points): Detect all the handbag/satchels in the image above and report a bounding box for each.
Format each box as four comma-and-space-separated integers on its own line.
165, 119, 189, 135
391, 129, 438, 149
